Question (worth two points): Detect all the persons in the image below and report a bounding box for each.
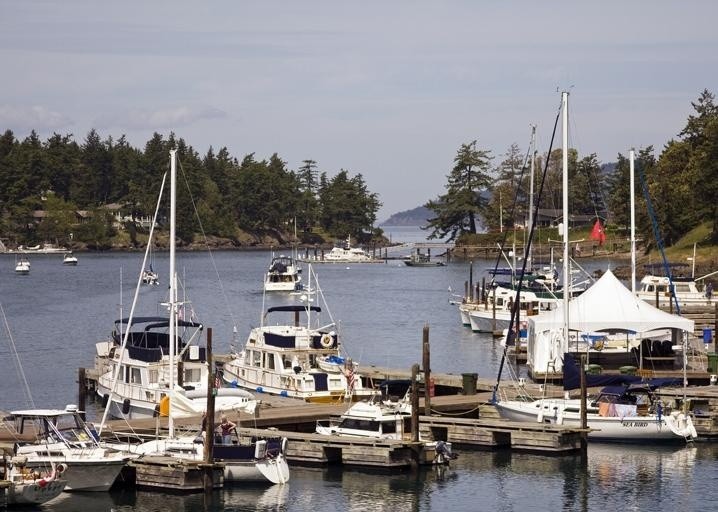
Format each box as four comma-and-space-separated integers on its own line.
217, 411, 237, 443
199, 409, 208, 441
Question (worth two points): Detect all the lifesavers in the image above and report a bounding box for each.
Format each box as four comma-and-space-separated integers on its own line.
320, 333, 334, 348
668, 412, 695, 437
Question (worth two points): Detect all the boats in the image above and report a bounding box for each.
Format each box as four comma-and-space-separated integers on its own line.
403, 246, 448, 267
221, 262, 384, 399
138, 263, 162, 288
262, 254, 304, 293
97, 317, 260, 421
15, 258, 30, 275
297, 233, 385, 263
62, 250, 78, 265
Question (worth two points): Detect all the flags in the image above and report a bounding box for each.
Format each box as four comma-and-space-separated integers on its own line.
592, 220, 605, 244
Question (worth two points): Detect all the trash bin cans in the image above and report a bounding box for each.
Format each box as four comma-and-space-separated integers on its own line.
619, 366, 637, 376
707, 352, 718, 374
462, 373, 478, 395
703, 329, 711, 343
586, 364, 601, 375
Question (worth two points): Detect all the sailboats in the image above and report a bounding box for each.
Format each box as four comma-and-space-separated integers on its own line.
101, 150, 293, 490
494, 91, 698, 440
